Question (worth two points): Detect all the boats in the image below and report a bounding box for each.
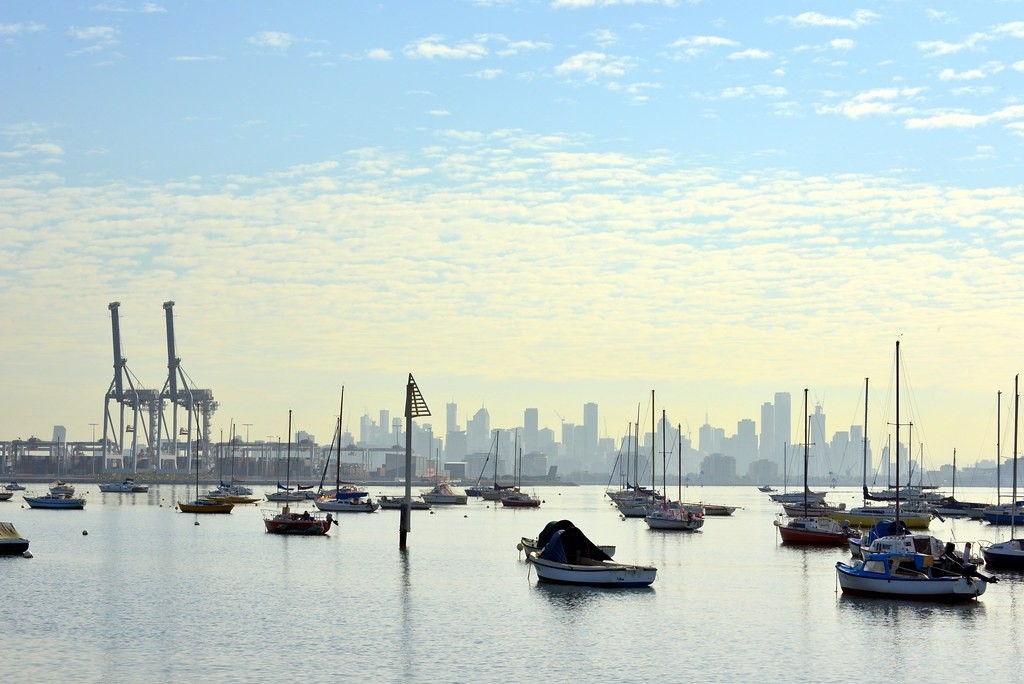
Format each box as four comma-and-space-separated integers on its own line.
0, 522, 30, 555
0, 484, 14, 500
517, 519, 616, 561
49, 485, 75, 496
5, 481, 26, 490
528, 528, 657, 587
420, 449, 467, 504
376, 496, 432, 510
757, 484, 778, 492
98, 478, 149, 492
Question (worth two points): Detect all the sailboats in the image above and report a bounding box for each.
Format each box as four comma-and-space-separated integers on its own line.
501, 448, 541, 506
465, 427, 519, 506
606, 391, 741, 531
768, 342, 1024, 602
263, 410, 338, 536
177, 403, 261, 513
265, 417, 379, 512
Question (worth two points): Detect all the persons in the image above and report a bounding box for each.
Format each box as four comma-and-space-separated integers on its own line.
939, 542, 971, 575
300, 511, 310, 521
46, 493, 50, 496
